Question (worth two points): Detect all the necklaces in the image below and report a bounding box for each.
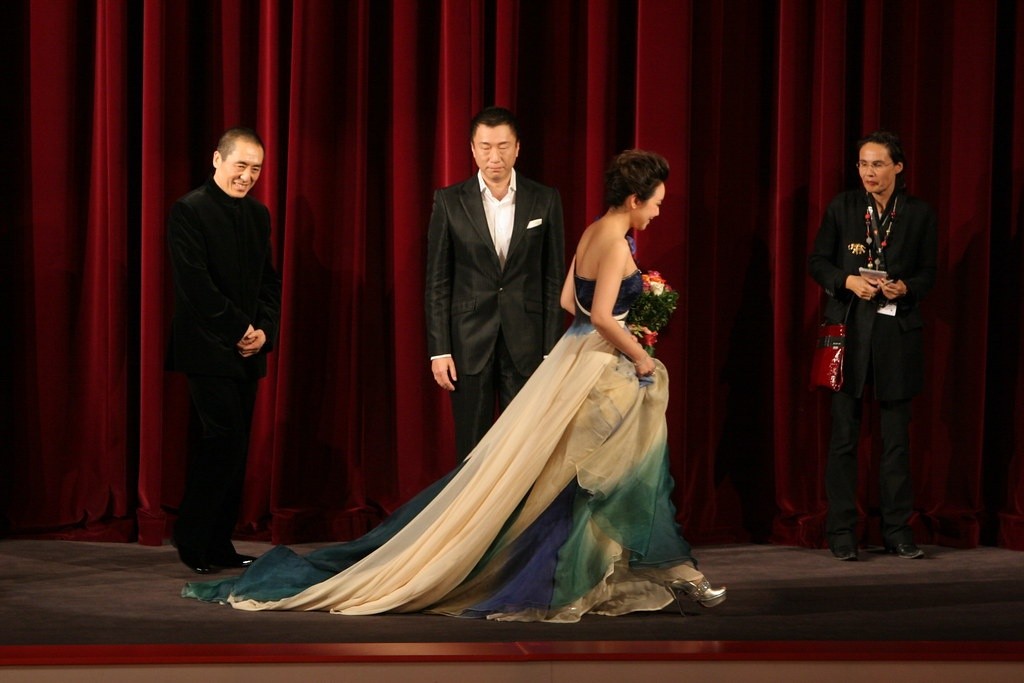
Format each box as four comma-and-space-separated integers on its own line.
865, 192, 897, 269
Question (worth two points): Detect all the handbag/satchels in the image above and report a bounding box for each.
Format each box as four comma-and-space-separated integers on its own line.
809, 321, 847, 391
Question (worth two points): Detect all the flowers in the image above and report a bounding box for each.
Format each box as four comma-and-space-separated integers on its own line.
628, 266, 680, 353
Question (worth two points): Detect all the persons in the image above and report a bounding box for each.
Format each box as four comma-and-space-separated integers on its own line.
170, 128, 283, 574
181, 149, 727, 624
422, 102, 567, 462
805, 134, 926, 561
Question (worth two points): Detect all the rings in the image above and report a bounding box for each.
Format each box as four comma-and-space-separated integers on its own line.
652, 372, 655, 375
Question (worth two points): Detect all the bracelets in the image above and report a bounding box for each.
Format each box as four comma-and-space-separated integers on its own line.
634, 350, 648, 367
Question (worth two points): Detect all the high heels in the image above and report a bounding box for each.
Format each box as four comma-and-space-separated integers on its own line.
663, 573, 728, 614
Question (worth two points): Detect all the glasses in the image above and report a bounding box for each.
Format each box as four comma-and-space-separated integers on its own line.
856, 160, 894, 170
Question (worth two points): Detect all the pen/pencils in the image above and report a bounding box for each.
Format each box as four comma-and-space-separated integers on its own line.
874, 280, 896, 292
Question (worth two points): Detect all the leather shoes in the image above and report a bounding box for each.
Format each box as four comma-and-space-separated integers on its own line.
194, 561, 222, 575
833, 547, 857, 561
887, 544, 924, 560
215, 553, 258, 569
